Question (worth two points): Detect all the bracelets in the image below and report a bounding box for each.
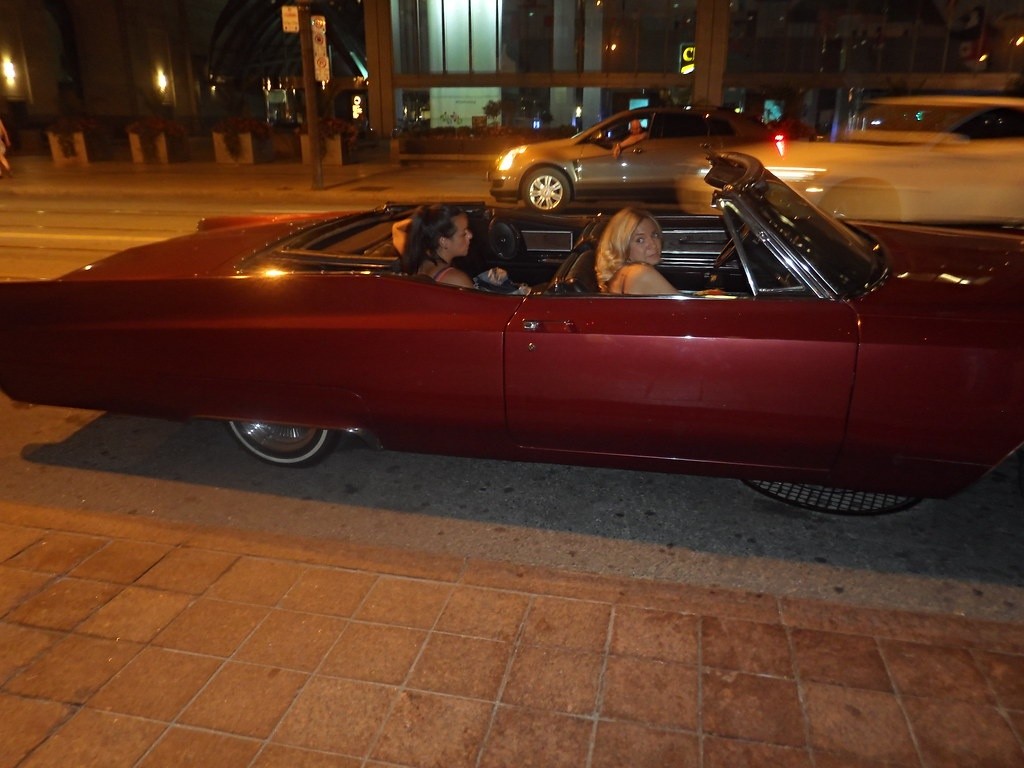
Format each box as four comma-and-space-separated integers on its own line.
617, 142, 622, 149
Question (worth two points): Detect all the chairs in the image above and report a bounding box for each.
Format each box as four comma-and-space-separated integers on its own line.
548, 249, 599, 294
572, 213, 608, 251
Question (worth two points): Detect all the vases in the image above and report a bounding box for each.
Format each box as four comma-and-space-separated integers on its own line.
128, 132, 173, 164
46, 131, 95, 162
298, 134, 350, 166
211, 131, 265, 165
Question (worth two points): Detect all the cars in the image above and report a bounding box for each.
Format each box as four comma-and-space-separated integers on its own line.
492, 101, 796, 206
1, 146, 1023, 492
678, 93, 1023, 231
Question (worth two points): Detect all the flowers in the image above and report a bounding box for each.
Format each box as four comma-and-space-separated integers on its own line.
45, 119, 114, 162
295, 115, 360, 161
210, 115, 274, 165
125, 114, 189, 165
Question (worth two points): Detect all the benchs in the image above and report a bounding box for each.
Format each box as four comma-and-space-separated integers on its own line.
361, 237, 548, 295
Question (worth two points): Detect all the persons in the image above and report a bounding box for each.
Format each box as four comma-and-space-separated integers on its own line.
594, 206, 679, 294
391, 201, 476, 288
0, 115, 15, 179
612, 119, 649, 160
